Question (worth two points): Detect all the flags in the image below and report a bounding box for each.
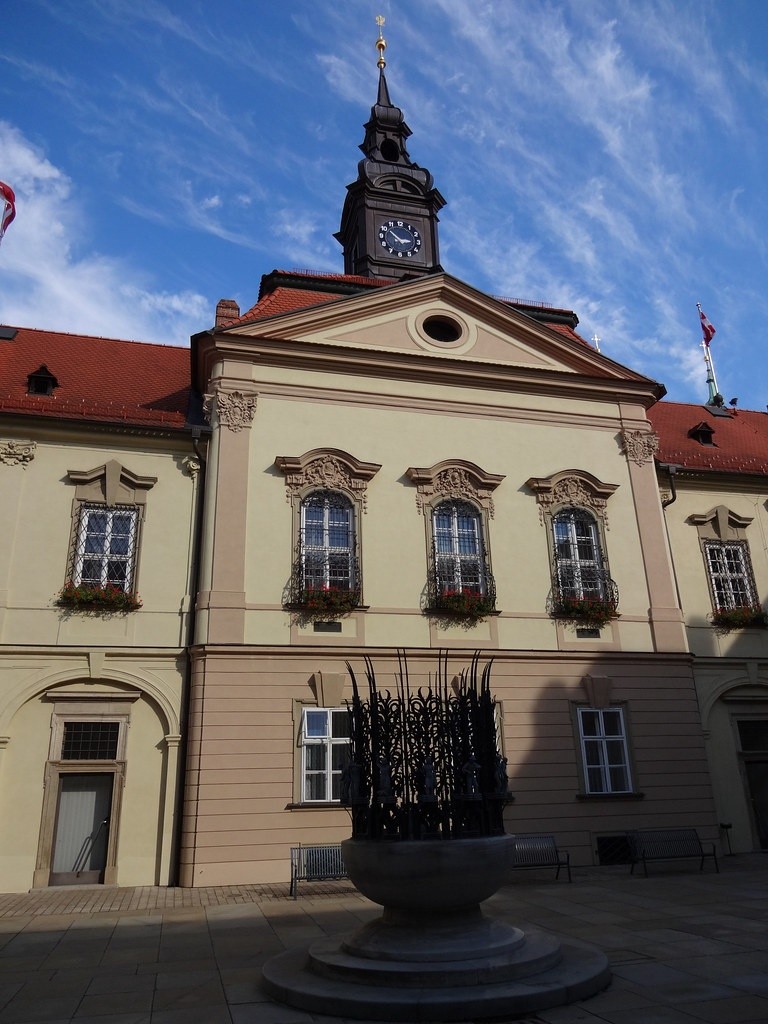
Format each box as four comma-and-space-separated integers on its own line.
0, 182, 16, 241
699, 310, 716, 347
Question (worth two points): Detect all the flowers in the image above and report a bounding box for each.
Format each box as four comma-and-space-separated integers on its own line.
705, 600, 768, 634
553, 594, 622, 630
53, 579, 144, 610
298, 580, 360, 615
427, 588, 495, 625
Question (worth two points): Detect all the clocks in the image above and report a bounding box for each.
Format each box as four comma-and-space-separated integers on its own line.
379, 220, 422, 258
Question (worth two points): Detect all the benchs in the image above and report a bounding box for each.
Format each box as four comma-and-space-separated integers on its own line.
512, 835, 572, 884
289, 847, 349, 901
624, 828, 719, 878
